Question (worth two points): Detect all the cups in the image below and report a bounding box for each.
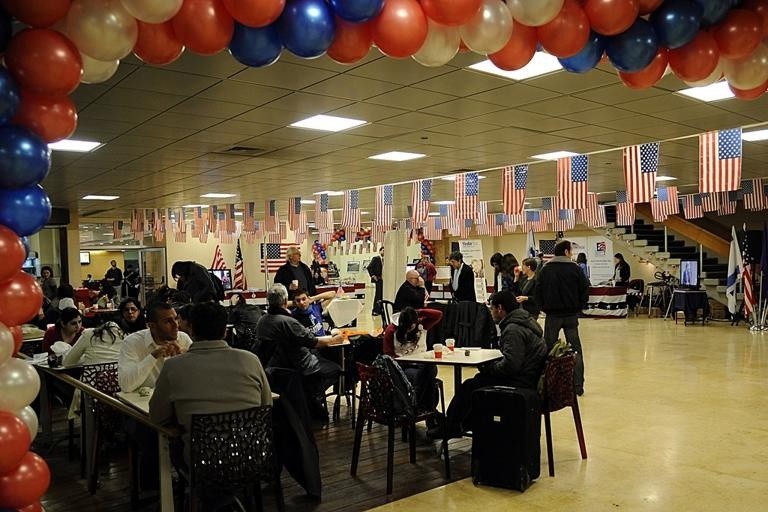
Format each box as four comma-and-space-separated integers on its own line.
292, 280, 299, 288
433, 344, 443, 359
514, 265, 522, 274
444, 339, 455, 353
139, 386, 152, 397
464, 348, 472, 357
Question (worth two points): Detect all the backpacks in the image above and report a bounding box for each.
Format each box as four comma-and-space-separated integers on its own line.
373, 354, 418, 415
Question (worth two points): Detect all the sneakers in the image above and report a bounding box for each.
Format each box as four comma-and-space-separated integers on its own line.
576, 387, 584, 396
426, 418, 464, 439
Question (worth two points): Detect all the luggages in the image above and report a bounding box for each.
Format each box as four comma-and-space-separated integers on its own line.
470, 384, 539, 494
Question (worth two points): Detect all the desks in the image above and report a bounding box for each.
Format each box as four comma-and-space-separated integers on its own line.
637, 281, 664, 318
664, 288, 710, 326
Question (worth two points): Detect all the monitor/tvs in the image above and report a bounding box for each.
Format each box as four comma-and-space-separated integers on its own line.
80, 252, 90, 264
207, 268, 232, 290
680, 259, 700, 290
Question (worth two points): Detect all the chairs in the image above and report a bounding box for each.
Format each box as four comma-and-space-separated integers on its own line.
626, 279, 645, 318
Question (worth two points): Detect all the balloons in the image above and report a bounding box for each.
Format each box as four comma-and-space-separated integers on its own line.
0, 1, 138, 512
311, 237, 327, 265
138, 0, 768, 99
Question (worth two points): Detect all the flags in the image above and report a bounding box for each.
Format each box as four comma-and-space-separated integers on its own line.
622, 141, 661, 204
260, 242, 301, 272
556, 155, 589, 210
726, 225, 744, 315
234, 238, 248, 291
698, 126, 742, 194
502, 164, 528, 215
211, 245, 227, 269
760, 220, 767, 300
740, 228, 759, 319
110, 171, 768, 251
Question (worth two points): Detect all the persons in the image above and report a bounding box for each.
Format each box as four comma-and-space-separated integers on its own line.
227, 293, 262, 349
683, 261, 691, 285
147, 302, 276, 511
608, 252, 630, 287
254, 283, 344, 426
392, 270, 430, 312
290, 287, 337, 337
273, 247, 318, 309
415, 250, 591, 327
366, 247, 386, 317
426, 289, 548, 442
117, 299, 193, 392
172, 260, 218, 304
535, 239, 589, 397
392, 306, 447, 429
